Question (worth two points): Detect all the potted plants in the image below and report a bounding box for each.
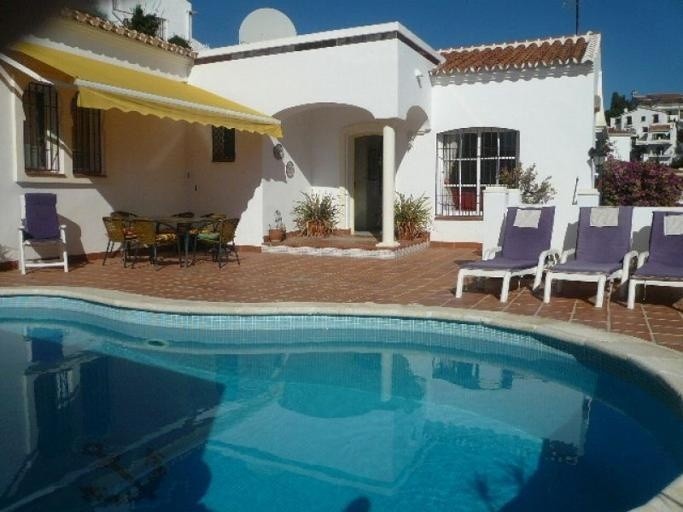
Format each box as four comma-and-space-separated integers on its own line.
394, 190, 434, 242
266, 208, 287, 241
289, 189, 346, 240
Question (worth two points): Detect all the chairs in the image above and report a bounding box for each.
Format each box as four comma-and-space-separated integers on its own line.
17, 192, 70, 276
453, 202, 683, 310
102, 209, 241, 271
